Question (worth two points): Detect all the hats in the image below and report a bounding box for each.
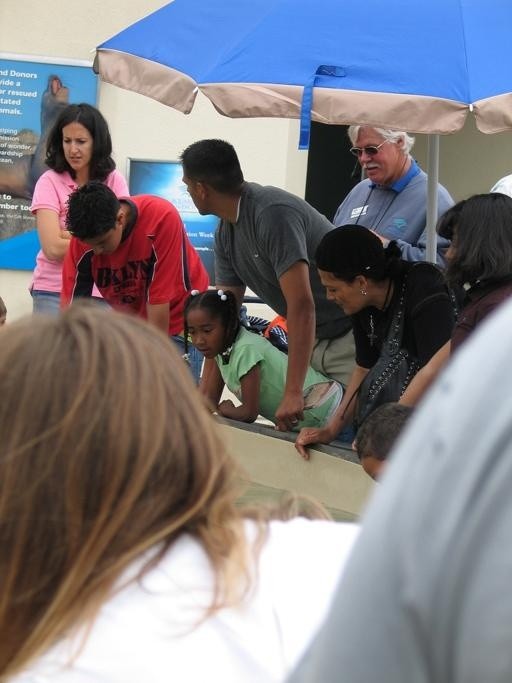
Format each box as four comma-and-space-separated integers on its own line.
317, 224, 384, 272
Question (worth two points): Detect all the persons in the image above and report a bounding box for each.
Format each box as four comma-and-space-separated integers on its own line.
50, 180, 212, 386
283, 285, 511, 681
29, 102, 132, 314
2, 297, 363, 683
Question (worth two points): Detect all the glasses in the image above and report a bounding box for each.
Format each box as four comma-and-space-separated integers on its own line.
348, 139, 390, 158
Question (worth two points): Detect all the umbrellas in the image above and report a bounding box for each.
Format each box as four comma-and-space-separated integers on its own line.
88, 0, 512, 274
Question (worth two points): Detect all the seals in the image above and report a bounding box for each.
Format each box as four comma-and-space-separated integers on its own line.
0, 75, 69, 201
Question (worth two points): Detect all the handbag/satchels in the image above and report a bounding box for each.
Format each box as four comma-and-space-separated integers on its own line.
351, 261, 458, 435
